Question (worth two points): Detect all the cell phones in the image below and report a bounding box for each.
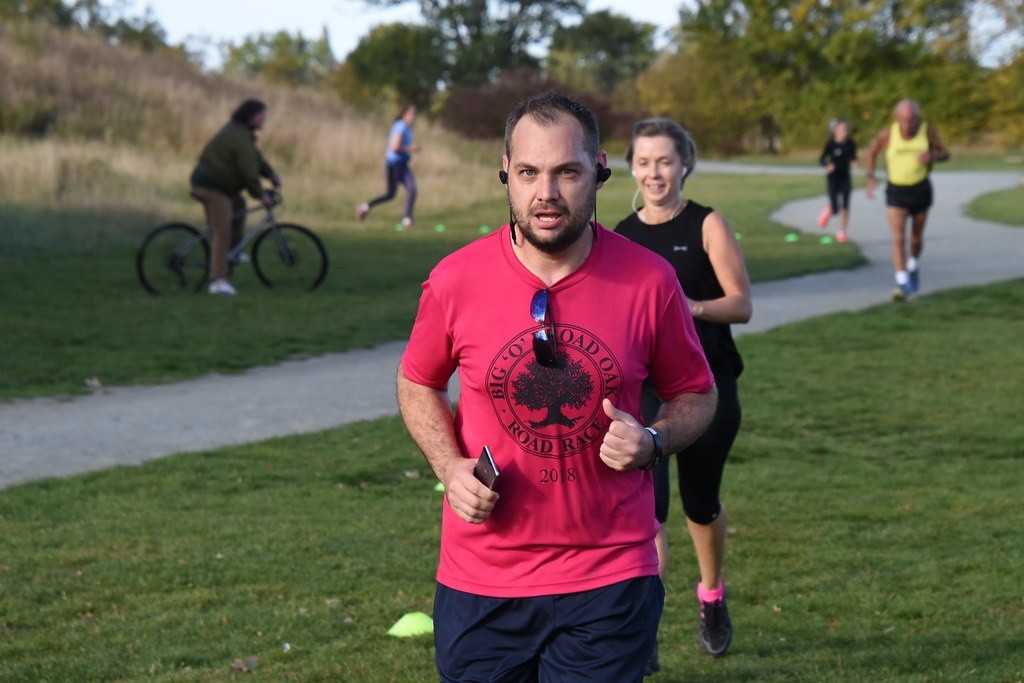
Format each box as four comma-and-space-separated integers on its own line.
474, 445, 499, 491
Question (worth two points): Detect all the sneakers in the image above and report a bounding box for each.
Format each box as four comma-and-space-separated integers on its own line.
696, 578, 732, 656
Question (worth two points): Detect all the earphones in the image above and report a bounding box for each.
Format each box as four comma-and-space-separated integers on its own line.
596, 163, 612, 183
499, 170, 508, 185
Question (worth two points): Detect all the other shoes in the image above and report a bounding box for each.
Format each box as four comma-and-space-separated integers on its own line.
890, 283, 907, 301
234, 253, 250, 263
356, 203, 369, 221
402, 217, 413, 227
906, 266, 919, 291
208, 280, 237, 295
837, 231, 847, 242
820, 211, 831, 227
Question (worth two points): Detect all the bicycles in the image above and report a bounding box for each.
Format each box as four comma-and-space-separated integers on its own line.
136, 189, 328, 295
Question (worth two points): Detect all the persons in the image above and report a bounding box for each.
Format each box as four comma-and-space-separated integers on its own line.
190, 99, 281, 295
610, 119, 752, 657
866, 99, 950, 301
396, 91, 718, 683
354, 103, 420, 228
818, 121, 858, 242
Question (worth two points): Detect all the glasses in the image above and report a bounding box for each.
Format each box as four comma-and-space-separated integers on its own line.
530, 289, 560, 369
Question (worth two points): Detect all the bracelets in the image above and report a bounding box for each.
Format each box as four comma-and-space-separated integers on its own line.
640, 427, 663, 471
